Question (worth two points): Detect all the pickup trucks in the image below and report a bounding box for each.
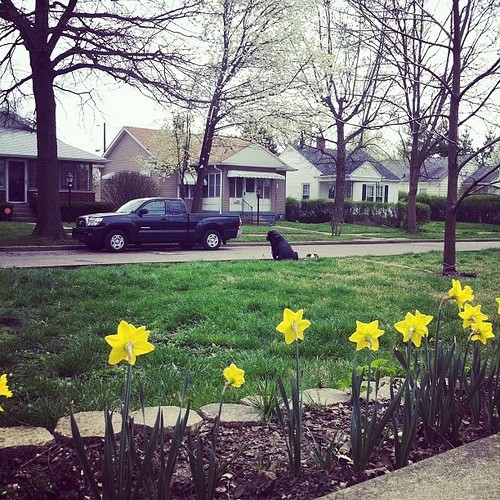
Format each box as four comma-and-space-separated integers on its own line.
72, 197, 240, 249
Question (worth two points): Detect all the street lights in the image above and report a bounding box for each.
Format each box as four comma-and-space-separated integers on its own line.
65, 172, 75, 224
256, 187, 261, 226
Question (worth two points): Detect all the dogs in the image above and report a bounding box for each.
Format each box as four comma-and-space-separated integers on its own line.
266, 230, 299, 262
